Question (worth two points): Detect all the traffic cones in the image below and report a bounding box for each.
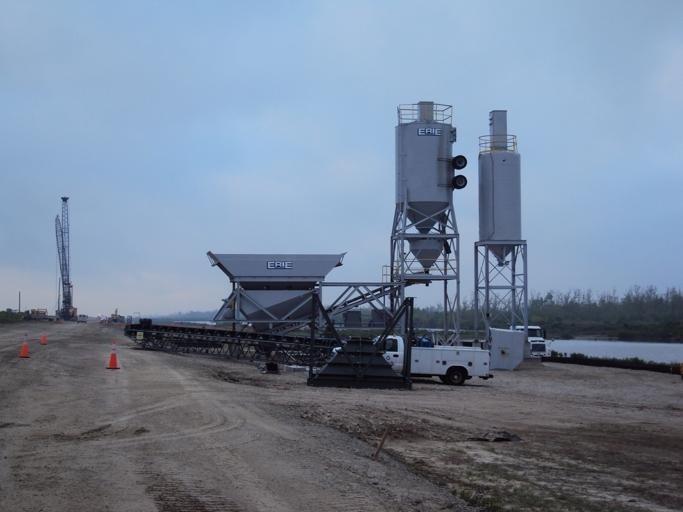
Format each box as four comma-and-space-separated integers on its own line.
106, 346, 119, 369
19, 332, 30, 358
40, 329, 47, 345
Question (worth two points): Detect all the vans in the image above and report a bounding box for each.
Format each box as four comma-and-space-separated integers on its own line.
77, 313, 88, 324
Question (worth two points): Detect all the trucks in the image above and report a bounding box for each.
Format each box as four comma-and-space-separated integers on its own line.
332, 335, 489, 386
508, 325, 551, 362
